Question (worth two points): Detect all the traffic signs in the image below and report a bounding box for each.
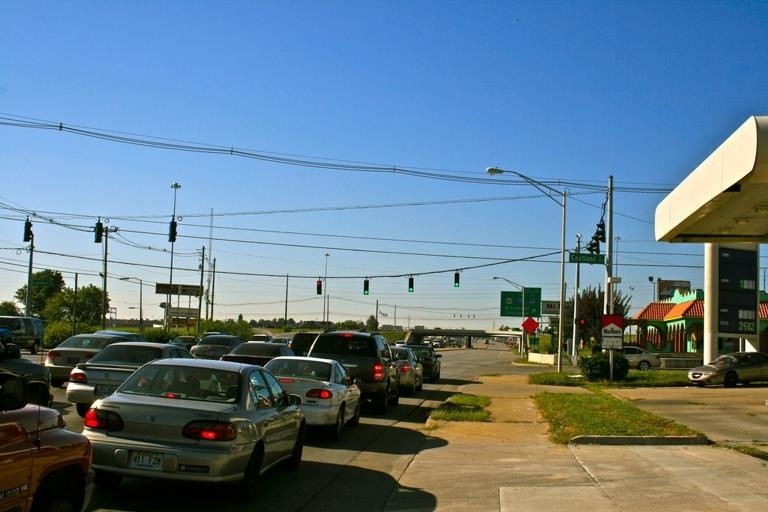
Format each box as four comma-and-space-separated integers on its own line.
523, 287, 541, 317
569, 252, 605, 265
500, 290, 522, 316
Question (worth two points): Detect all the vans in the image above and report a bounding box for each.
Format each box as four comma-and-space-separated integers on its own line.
0, 316, 44, 354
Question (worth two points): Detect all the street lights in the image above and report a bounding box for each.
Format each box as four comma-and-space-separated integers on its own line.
119, 277, 142, 330
486, 167, 567, 374
614, 236, 621, 304
164, 182, 182, 334
492, 276, 526, 360
628, 285, 635, 343
129, 306, 144, 328
323, 253, 330, 330
649, 276, 662, 302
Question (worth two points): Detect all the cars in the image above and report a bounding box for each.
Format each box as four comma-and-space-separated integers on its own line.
0, 372, 96, 511
0, 340, 53, 408
687, 352, 768, 388
588, 346, 661, 371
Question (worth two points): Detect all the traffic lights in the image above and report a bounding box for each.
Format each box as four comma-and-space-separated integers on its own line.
596, 222, 606, 243
317, 280, 321, 294
409, 277, 414, 293
454, 272, 460, 288
23, 221, 33, 242
586, 243, 593, 253
364, 279, 370, 295
591, 234, 600, 256
